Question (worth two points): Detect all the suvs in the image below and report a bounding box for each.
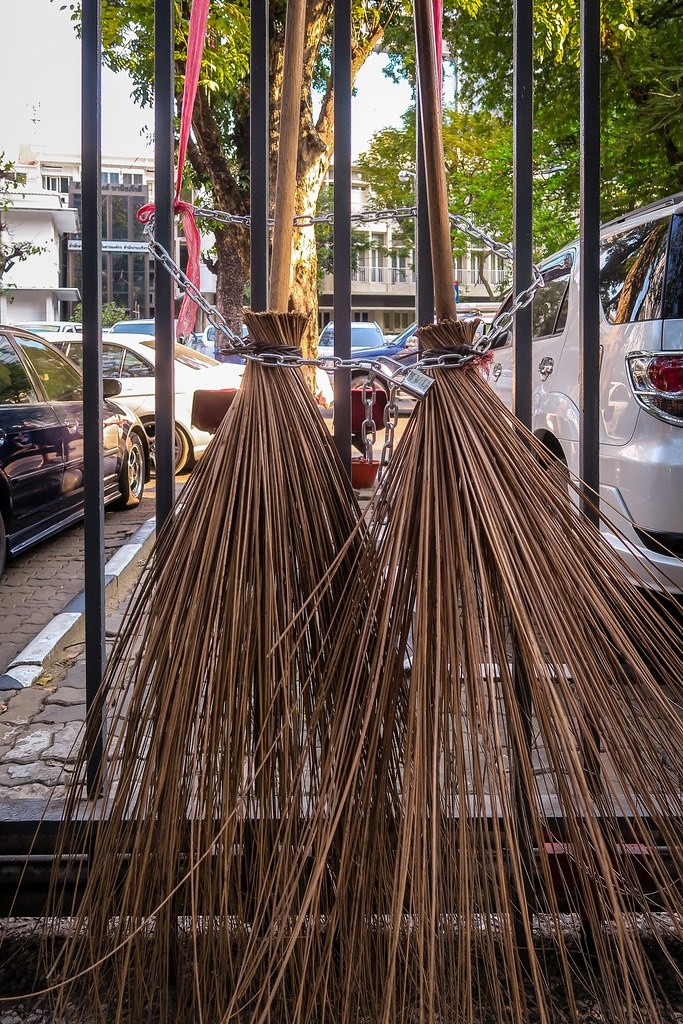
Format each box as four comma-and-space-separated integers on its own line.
477, 189, 683, 596
0, 324, 151, 578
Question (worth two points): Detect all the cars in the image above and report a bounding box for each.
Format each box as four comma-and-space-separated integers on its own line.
352, 312, 494, 408
31, 331, 247, 481
314, 320, 385, 376
201, 324, 250, 365
10, 316, 204, 359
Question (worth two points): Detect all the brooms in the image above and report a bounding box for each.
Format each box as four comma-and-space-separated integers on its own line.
0, 0, 683, 1024
